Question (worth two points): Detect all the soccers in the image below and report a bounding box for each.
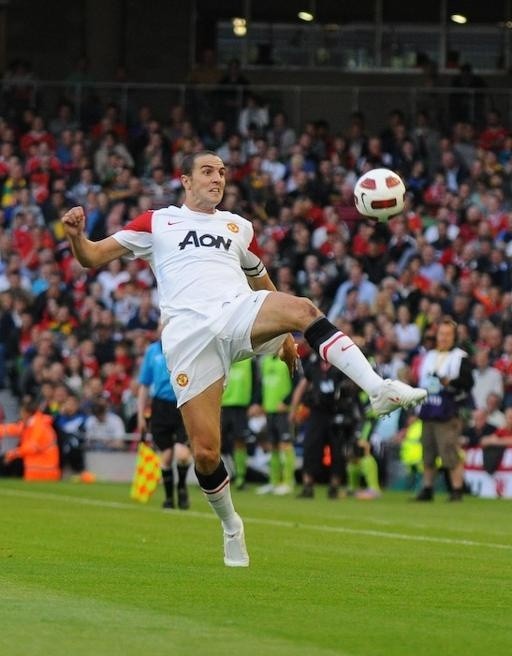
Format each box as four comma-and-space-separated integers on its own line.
354, 168, 406, 221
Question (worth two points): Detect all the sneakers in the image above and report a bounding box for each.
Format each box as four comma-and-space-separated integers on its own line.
221, 512, 250, 567
177, 483, 189, 508
368, 378, 428, 422
232, 472, 467, 502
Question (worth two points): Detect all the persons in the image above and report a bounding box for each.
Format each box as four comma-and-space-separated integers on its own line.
57, 151, 429, 568
2, 26, 512, 513
